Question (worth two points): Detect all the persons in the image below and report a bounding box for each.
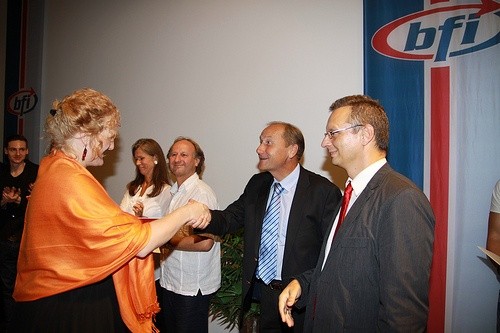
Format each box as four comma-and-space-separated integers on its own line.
0, 135, 40, 304
486, 180, 500, 333
279, 95, 435, 333
119, 138, 172, 333
12, 88, 210, 333
158, 136, 221, 333
184, 121, 342, 333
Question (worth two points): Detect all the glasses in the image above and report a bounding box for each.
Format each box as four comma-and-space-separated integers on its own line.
325, 124, 365, 140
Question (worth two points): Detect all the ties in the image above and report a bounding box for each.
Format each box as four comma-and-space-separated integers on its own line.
258, 183, 284, 286
333, 183, 353, 243
